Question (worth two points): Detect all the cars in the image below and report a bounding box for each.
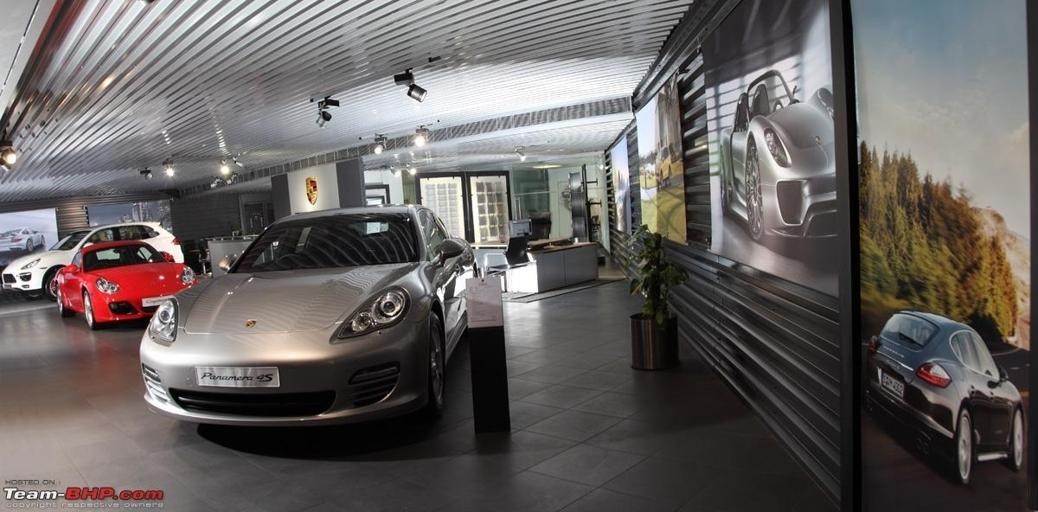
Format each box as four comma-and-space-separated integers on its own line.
640, 143, 679, 190
867, 310, 1027, 486
0, 227, 45, 254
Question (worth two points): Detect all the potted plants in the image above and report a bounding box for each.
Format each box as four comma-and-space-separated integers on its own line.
621, 222, 690, 372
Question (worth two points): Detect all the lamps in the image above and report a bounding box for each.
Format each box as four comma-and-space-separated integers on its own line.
314, 95, 339, 129
372, 126, 433, 155
394, 66, 429, 103
514, 145, 527, 161
390, 162, 417, 175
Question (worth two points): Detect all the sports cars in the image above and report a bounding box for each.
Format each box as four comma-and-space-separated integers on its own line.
719, 70, 839, 243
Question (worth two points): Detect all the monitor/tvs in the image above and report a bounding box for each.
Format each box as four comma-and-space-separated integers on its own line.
509, 219, 532, 237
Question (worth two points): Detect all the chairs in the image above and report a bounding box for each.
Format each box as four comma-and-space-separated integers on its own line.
90, 229, 143, 242
318, 223, 413, 265
84, 251, 141, 269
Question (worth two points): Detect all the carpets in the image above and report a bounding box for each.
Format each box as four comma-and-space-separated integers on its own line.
500, 275, 628, 303
